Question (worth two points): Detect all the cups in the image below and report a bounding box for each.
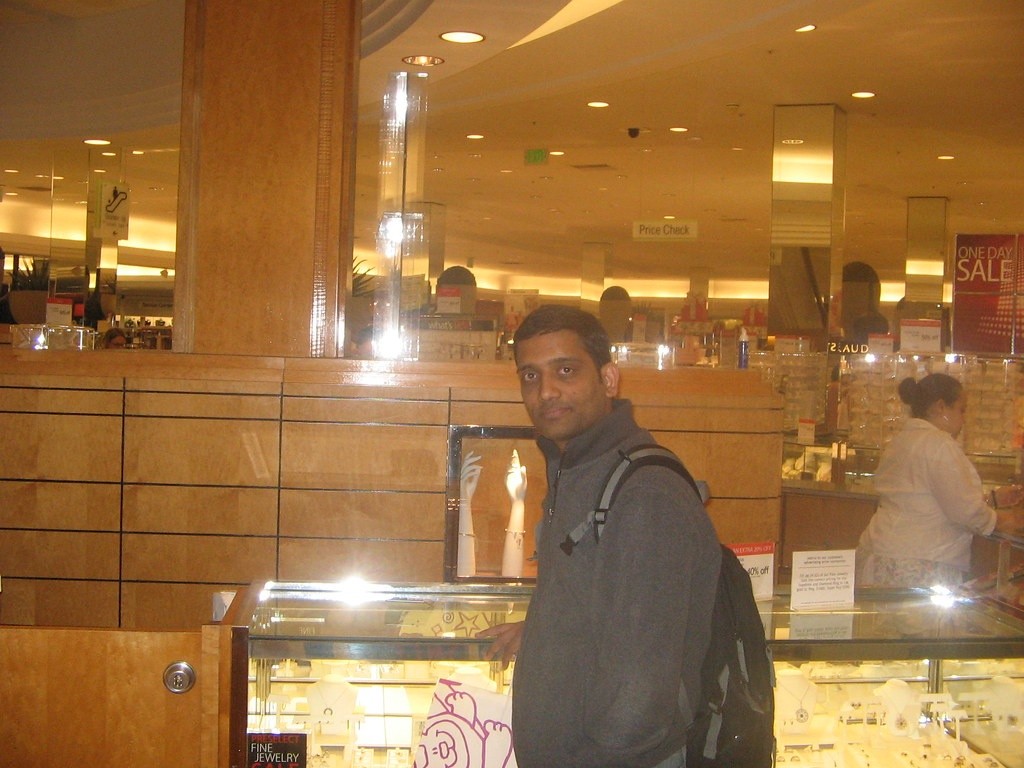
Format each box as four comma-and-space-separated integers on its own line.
656, 345, 676, 370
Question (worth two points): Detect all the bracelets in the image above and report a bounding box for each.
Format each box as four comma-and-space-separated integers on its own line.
991, 489, 998, 510
459, 531, 474, 538
504, 528, 526, 535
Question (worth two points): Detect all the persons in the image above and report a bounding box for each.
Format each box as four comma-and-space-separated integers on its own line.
503, 446, 528, 576
103, 328, 126, 349
473, 303, 723, 767
854, 373, 1024, 589
457, 451, 484, 577
595, 286, 657, 345
401, 265, 493, 331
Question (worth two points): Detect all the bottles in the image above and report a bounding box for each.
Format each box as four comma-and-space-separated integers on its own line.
610, 344, 632, 365
738, 329, 750, 368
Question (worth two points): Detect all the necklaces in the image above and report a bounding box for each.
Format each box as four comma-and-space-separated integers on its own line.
781, 685, 811, 722
883, 692, 912, 730
315, 682, 341, 717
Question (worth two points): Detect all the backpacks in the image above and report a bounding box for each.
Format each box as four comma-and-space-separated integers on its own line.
561, 444, 775, 768
